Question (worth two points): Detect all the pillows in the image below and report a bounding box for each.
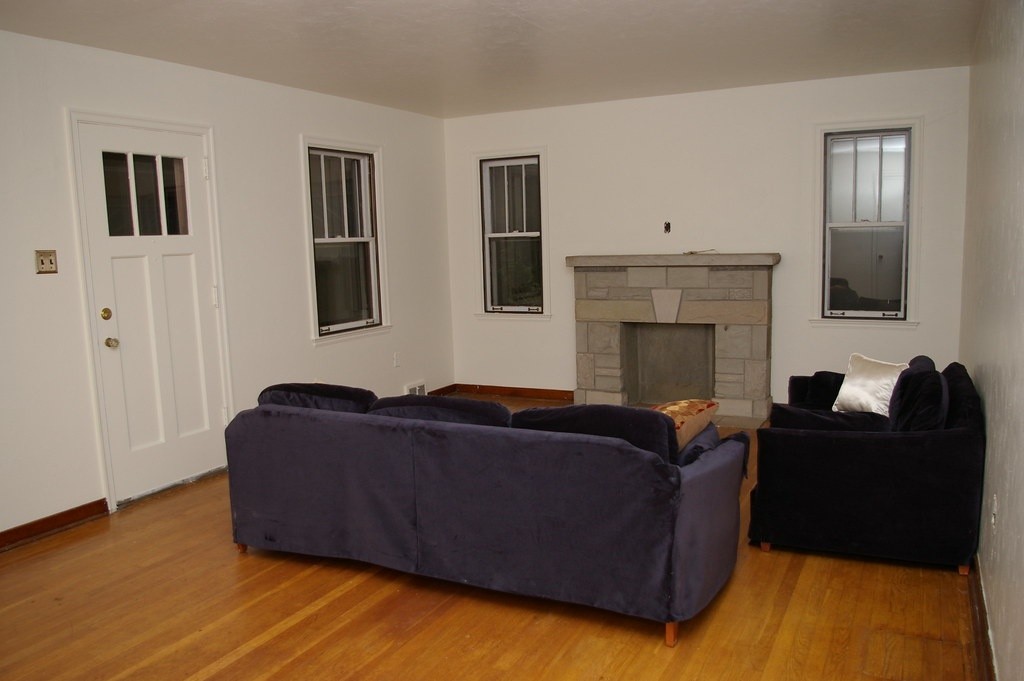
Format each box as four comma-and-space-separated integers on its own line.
511, 404, 678, 465
258, 382, 377, 416
768, 400, 891, 431
889, 355, 935, 431
679, 422, 720, 468
904, 370, 948, 431
832, 352, 909, 419
373, 394, 511, 428
651, 400, 720, 449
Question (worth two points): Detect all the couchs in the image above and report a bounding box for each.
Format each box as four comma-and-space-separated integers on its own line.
223, 383, 752, 647
748, 355, 986, 576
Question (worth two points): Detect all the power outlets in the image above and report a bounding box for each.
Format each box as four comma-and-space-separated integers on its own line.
394, 352, 400, 366
405, 381, 428, 396
